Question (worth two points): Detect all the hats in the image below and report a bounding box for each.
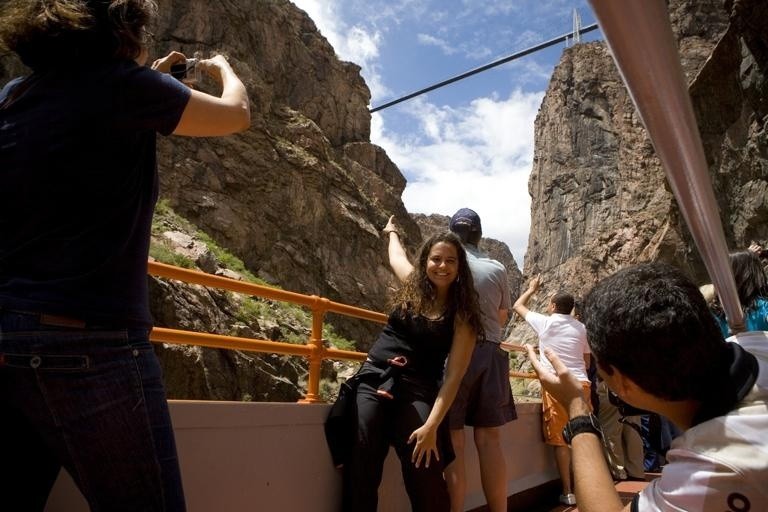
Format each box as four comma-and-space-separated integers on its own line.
450, 207, 483, 232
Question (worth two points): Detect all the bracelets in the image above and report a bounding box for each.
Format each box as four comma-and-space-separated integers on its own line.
386, 230, 397, 236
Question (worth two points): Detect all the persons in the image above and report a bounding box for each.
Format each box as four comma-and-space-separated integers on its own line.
341, 214, 487, 511
441, 207, 516, 511
0, 0, 251, 511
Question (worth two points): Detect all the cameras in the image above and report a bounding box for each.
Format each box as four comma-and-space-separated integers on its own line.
171, 58, 203, 84
